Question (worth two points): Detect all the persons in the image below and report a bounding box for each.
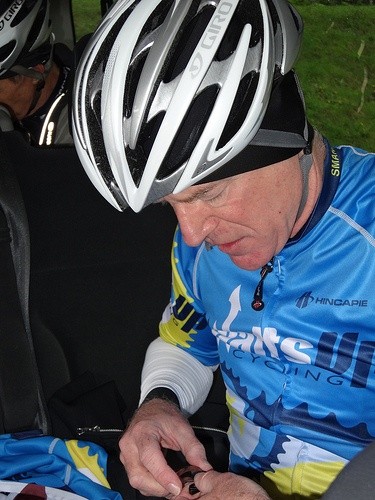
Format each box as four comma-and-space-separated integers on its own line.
66, 1, 373, 499
0, 0, 81, 146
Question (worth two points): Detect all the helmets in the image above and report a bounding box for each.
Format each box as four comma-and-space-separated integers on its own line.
1, 0, 51, 79
70, 0, 304, 212
